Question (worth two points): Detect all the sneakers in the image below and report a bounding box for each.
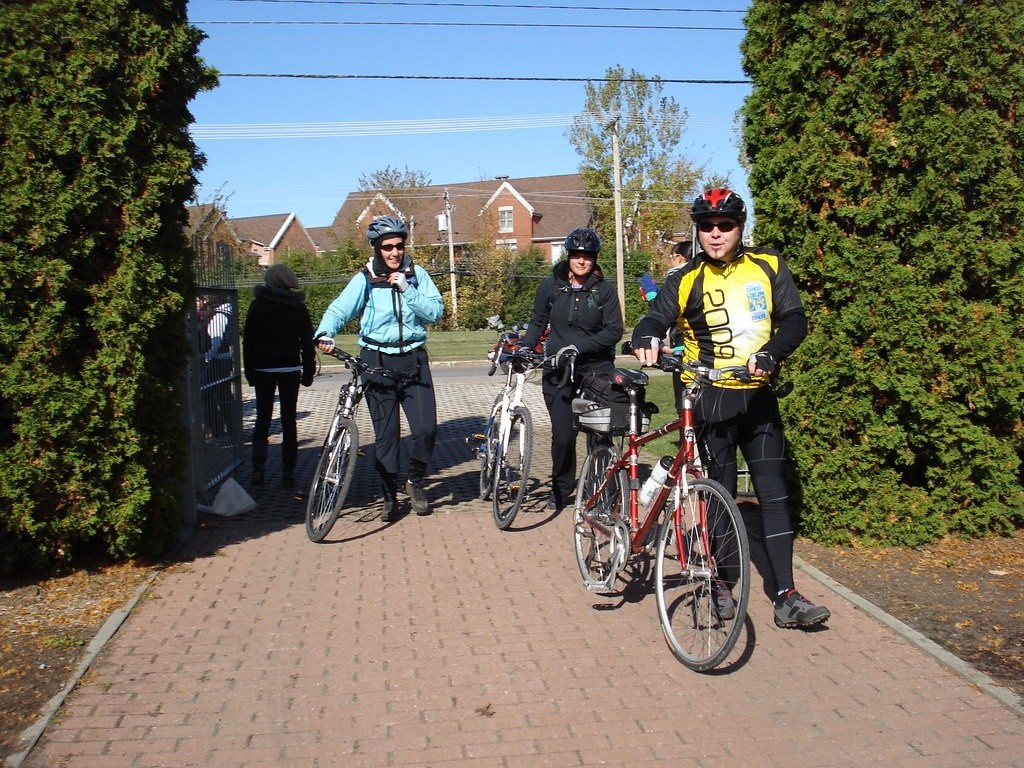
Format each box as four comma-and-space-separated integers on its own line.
403, 479, 429, 514
381, 496, 399, 521
706, 581, 734, 620
772, 589, 831, 629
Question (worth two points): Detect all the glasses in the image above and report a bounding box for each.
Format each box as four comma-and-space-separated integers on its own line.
696, 219, 737, 234
567, 250, 593, 258
381, 242, 404, 251
670, 252, 680, 258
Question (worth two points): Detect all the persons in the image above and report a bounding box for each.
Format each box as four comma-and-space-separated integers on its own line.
631, 189, 831, 632
639, 241, 693, 450
312, 216, 445, 521
242, 265, 317, 488
175, 289, 235, 445
512, 227, 624, 510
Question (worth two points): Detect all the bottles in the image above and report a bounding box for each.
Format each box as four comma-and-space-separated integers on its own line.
638, 455, 675, 509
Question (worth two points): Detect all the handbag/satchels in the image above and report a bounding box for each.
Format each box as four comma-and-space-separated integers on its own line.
574, 361, 661, 440
640, 274, 660, 301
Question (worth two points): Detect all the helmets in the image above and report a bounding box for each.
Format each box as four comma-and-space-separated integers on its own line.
690, 187, 747, 224
366, 216, 408, 247
564, 227, 602, 252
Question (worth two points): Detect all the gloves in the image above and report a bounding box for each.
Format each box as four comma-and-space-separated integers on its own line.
512, 346, 533, 373
555, 344, 579, 368
300, 359, 317, 387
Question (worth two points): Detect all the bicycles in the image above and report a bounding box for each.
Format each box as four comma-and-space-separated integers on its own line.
302, 332, 421, 545
466, 328, 579, 530
572, 346, 774, 672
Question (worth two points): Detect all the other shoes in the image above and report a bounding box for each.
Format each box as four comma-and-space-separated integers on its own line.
546, 481, 577, 509
282, 471, 296, 488
251, 470, 265, 486
